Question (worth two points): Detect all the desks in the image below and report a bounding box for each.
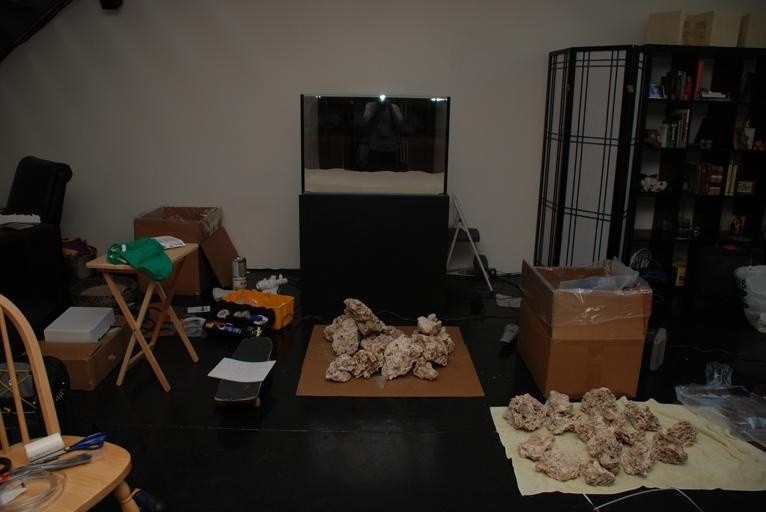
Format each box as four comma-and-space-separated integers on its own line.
87, 241, 202, 391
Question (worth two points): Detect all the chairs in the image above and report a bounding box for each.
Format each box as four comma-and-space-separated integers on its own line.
0, 293, 139, 512
0, 154, 74, 342
444, 196, 495, 297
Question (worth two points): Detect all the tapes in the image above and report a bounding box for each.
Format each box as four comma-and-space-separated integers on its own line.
216, 309, 229, 319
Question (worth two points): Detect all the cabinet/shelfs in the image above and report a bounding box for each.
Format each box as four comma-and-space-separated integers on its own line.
627, 43, 764, 353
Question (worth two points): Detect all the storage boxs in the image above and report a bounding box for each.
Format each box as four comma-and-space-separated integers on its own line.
645, 9, 766, 47
514, 252, 654, 400
131, 205, 223, 299
19, 308, 138, 391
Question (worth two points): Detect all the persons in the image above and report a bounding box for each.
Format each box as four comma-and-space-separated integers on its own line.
361, 97, 404, 173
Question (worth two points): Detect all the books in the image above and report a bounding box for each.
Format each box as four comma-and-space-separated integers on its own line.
647, 59, 766, 288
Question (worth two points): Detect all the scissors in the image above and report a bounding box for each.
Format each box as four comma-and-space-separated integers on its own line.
30, 431, 110, 465
